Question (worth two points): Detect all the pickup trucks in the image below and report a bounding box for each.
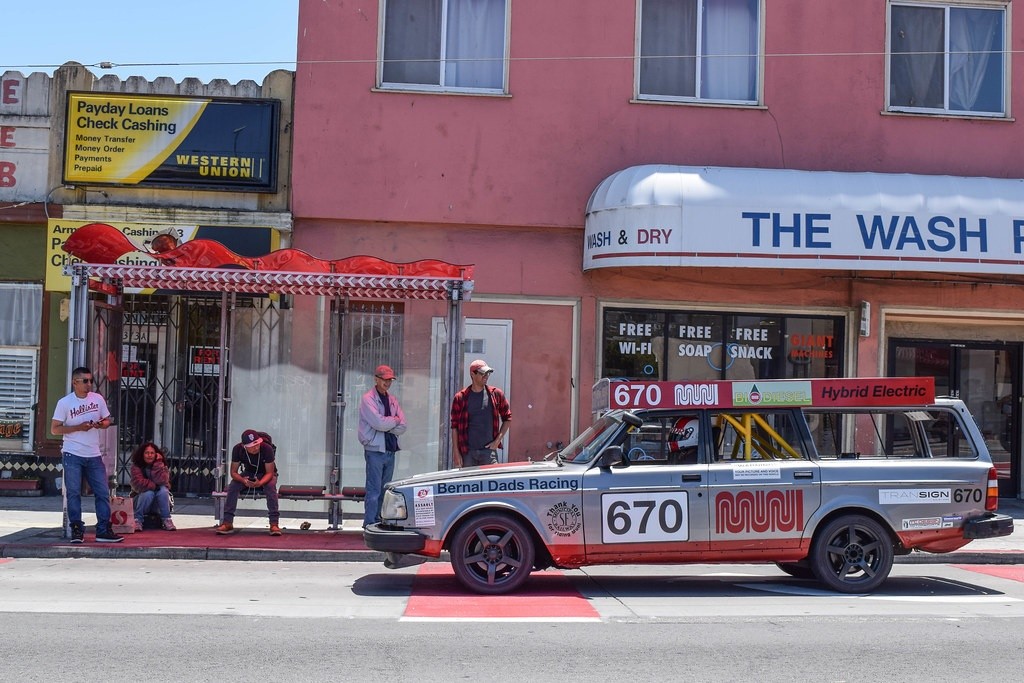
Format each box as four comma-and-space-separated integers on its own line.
362, 374, 1015, 595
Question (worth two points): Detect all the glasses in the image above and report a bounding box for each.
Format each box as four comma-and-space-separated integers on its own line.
376, 376, 395, 381
75, 378, 94, 384
474, 370, 490, 376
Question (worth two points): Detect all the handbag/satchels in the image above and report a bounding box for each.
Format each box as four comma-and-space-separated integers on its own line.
109, 492, 135, 534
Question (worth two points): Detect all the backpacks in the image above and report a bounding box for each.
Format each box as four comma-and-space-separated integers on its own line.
239, 431, 276, 472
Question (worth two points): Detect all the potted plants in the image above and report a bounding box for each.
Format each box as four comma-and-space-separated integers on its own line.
0, 475, 42, 490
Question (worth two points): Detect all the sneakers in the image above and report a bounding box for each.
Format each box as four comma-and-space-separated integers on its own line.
270, 523, 281, 535
134, 518, 142, 531
70, 521, 85, 543
162, 517, 176, 530
95, 522, 124, 542
216, 520, 234, 534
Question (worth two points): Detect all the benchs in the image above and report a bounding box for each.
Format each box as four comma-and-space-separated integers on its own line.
212, 485, 333, 527
338, 486, 367, 501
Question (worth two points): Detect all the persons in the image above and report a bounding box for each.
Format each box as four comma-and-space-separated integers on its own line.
50, 368, 124, 543
667, 417, 698, 462
450, 360, 512, 468
215, 429, 281, 535
129, 443, 176, 531
358, 366, 408, 536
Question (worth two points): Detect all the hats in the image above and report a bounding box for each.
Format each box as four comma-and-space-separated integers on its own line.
375, 366, 397, 381
470, 360, 495, 373
242, 430, 263, 447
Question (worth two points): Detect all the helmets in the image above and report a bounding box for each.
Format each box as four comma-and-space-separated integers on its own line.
666, 415, 699, 453
